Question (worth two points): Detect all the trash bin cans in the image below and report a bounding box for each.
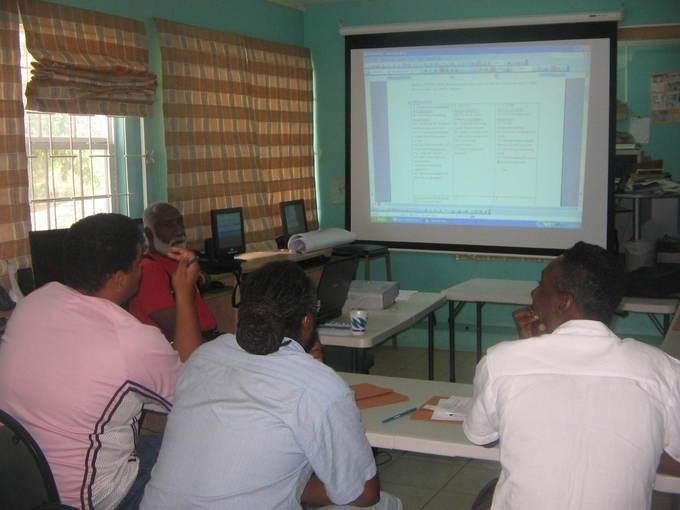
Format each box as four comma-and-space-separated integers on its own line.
625, 240, 649, 271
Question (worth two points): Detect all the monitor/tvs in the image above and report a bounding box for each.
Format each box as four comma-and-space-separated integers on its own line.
278, 199, 308, 238
210, 206, 247, 264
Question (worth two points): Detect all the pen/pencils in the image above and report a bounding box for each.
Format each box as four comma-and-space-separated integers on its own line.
187, 255, 200, 267
382, 406, 418, 423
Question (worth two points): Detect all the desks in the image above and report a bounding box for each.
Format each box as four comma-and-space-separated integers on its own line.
332, 373, 500, 462
653, 474, 677, 496
442, 279, 676, 384
614, 190, 680, 242
316, 290, 448, 381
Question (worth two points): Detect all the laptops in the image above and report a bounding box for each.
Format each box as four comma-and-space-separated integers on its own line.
310, 256, 358, 323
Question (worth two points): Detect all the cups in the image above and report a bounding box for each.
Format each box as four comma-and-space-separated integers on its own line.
349, 310, 369, 335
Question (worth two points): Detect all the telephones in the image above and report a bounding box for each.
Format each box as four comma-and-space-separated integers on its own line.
196, 250, 236, 274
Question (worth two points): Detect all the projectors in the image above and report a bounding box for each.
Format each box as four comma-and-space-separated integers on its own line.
347, 279, 400, 311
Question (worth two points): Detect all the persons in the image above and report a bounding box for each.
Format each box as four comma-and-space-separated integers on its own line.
138, 261, 403, 510
462, 241, 680, 510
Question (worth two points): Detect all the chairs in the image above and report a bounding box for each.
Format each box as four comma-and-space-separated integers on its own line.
0, 408, 74, 509
